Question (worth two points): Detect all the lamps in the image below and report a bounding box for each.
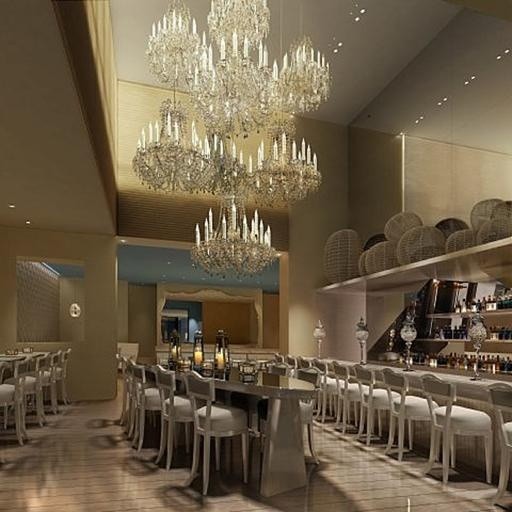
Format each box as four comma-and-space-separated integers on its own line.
133, 0, 334, 279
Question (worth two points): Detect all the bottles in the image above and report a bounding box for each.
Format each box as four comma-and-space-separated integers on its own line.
455, 293, 511, 313
431, 323, 510, 340
408, 351, 511, 372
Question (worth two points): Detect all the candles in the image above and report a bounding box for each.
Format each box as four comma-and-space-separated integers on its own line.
195, 352, 202, 365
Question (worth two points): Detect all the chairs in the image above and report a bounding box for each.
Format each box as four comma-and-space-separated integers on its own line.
273, 352, 512, 505
1, 347, 72, 446
115, 354, 324, 495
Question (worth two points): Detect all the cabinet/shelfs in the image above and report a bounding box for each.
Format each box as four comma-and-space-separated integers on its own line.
367, 308, 512, 375
